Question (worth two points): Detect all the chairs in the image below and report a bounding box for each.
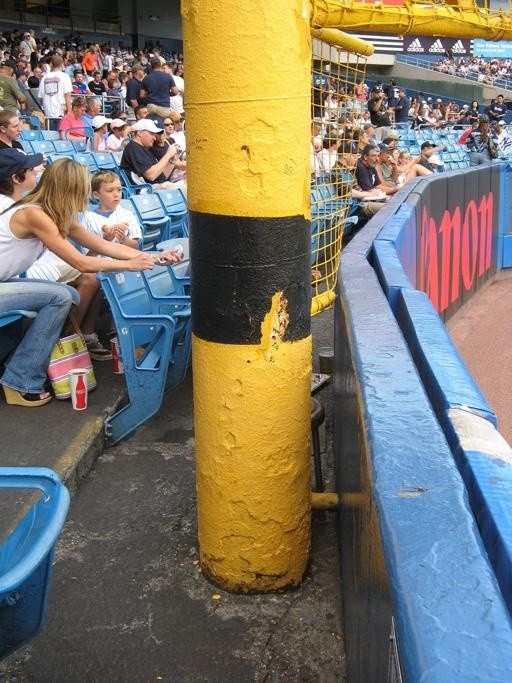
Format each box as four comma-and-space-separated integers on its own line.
1, 466, 74, 652
395, 120, 472, 170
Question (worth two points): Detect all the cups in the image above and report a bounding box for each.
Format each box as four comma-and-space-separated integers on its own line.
110, 337, 124, 375
67, 368, 90, 411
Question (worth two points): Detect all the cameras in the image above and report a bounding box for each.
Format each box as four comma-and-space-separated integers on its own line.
176, 144, 183, 153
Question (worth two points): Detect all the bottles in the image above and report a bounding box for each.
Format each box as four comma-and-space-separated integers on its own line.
113, 343, 119, 372
75, 376, 86, 409
380, 92, 387, 99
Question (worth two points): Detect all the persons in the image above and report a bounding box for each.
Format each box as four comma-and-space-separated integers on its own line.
2, 25, 185, 281
309, 55, 512, 280
1, 156, 186, 408
0, 147, 115, 362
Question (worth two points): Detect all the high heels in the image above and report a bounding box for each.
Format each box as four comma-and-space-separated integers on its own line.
1, 384, 51, 407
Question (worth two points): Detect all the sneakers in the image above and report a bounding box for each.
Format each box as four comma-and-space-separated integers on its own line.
84, 338, 112, 361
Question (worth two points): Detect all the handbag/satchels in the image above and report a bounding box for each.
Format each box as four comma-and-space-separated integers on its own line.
489, 138, 498, 160
47, 334, 98, 400
362, 191, 386, 202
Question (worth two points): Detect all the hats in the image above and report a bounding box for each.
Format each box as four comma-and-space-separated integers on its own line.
371, 86, 380, 92
480, 114, 488, 123
388, 134, 401, 140
364, 123, 377, 130
1, 148, 43, 172
0, 60, 16, 72
92, 113, 184, 136
421, 141, 435, 149
